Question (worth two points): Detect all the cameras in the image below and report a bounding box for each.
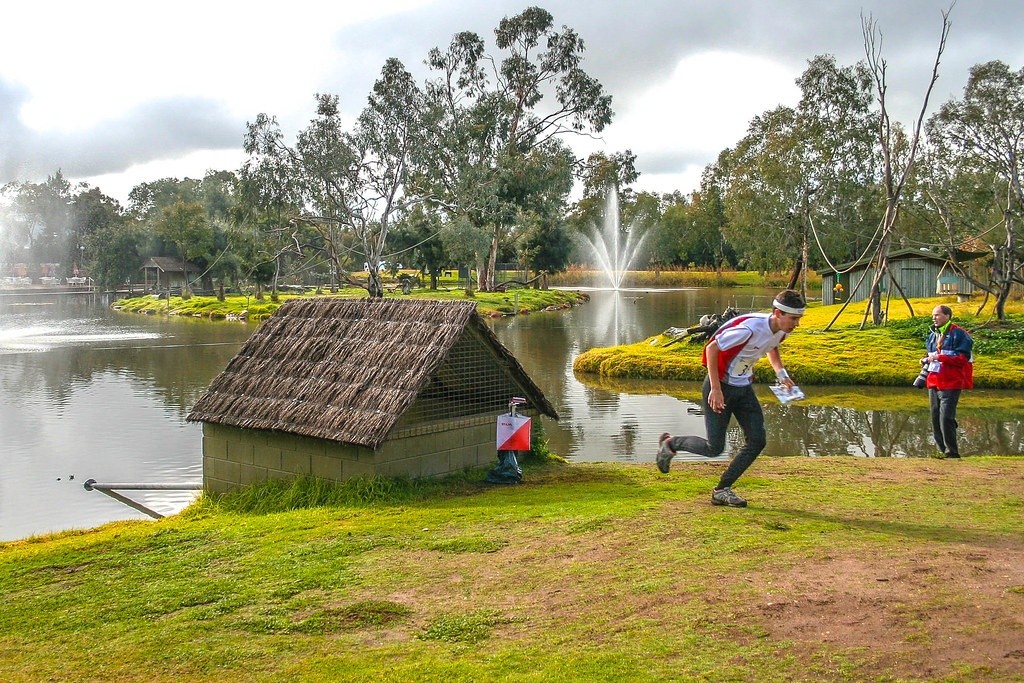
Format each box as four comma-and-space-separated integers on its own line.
913, 358, 931, 389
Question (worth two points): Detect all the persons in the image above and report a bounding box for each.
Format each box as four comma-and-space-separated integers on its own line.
921, 305, 974, 458
657, 291, 805, 508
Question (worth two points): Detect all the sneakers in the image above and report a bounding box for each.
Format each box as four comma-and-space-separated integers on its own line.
656, 432, 676, 473
710, 487, 748, 507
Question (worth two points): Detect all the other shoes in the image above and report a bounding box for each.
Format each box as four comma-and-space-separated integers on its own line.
939, 453, 961, 459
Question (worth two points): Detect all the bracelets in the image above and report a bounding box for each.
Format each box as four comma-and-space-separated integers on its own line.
775, 368, 789, 380
